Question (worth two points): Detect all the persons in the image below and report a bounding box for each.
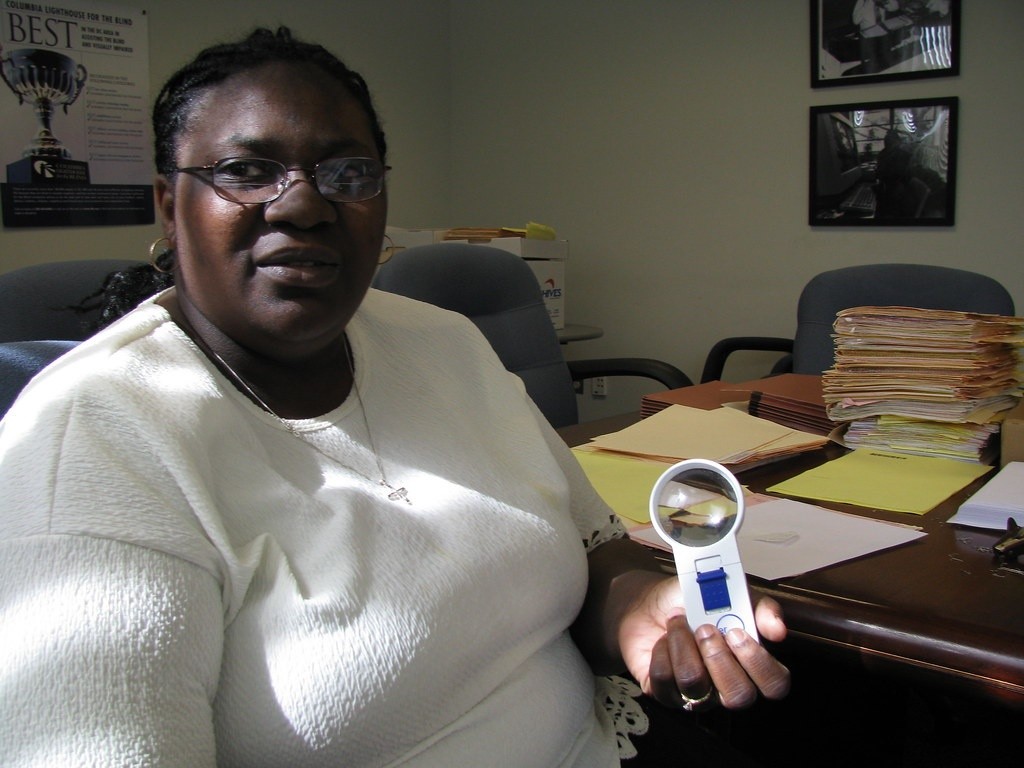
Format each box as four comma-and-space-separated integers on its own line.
0, 24, 791, 768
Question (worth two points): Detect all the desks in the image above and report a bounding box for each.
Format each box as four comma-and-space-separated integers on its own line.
553, 410, 1024, 713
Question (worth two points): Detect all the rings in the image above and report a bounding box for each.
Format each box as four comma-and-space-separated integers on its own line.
679, 685, 713, 710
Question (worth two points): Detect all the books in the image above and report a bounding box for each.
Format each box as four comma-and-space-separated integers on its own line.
822, 306, 1024, 465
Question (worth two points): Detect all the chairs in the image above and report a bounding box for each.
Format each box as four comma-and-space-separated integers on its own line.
0, 258, 154, 416
700, 262, 1014, 384
370, 243, 695, 429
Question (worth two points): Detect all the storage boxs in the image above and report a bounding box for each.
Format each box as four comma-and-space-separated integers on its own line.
385, 227, 568, 332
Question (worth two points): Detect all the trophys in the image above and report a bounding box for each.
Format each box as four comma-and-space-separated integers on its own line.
0, 46, 91, 184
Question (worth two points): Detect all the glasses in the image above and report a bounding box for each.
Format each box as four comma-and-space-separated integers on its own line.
158, 158, 394, 204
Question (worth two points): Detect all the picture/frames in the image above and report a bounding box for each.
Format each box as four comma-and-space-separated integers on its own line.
808, 96, 958, 227
809, 0, 960, 88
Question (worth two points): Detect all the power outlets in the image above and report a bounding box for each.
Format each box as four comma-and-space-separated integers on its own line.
591, 377, 607, 396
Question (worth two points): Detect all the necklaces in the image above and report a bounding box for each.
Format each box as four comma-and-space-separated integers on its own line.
214, 334, 413, 507
